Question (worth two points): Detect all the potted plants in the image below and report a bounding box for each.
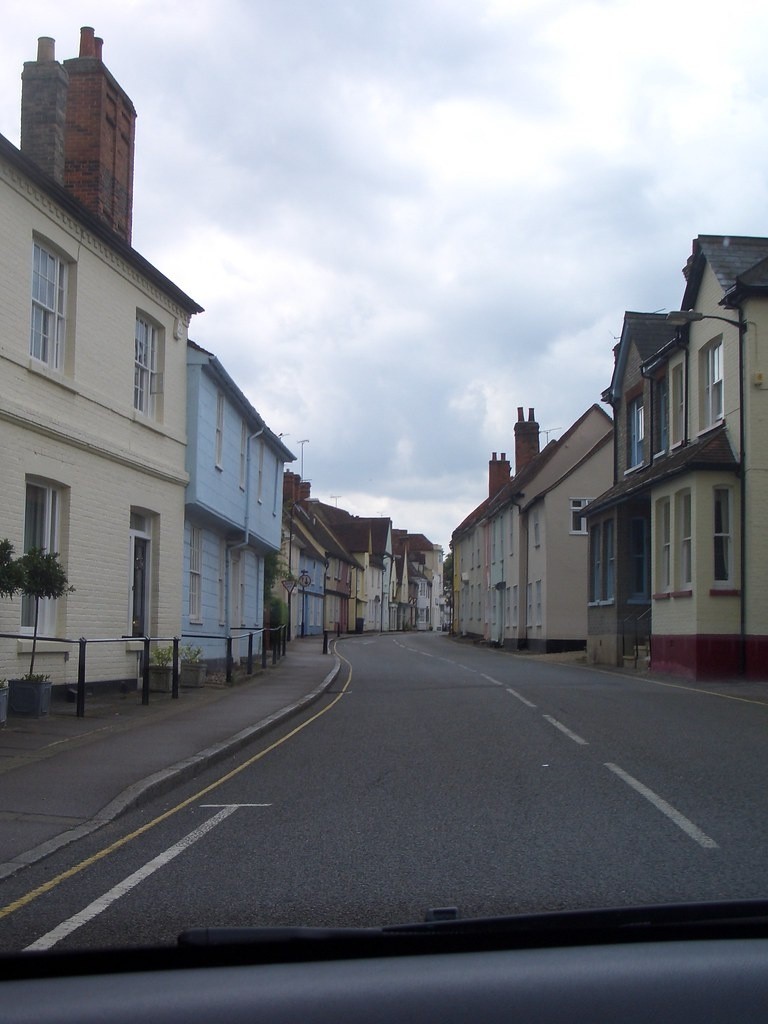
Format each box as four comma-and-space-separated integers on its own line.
6, 545, 77, 718
177, 642, 207, 687
0, 538, 24, 727
149, 646, 184, 693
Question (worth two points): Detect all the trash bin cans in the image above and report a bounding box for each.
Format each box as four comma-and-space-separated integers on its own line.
355, 617, 365, 634
